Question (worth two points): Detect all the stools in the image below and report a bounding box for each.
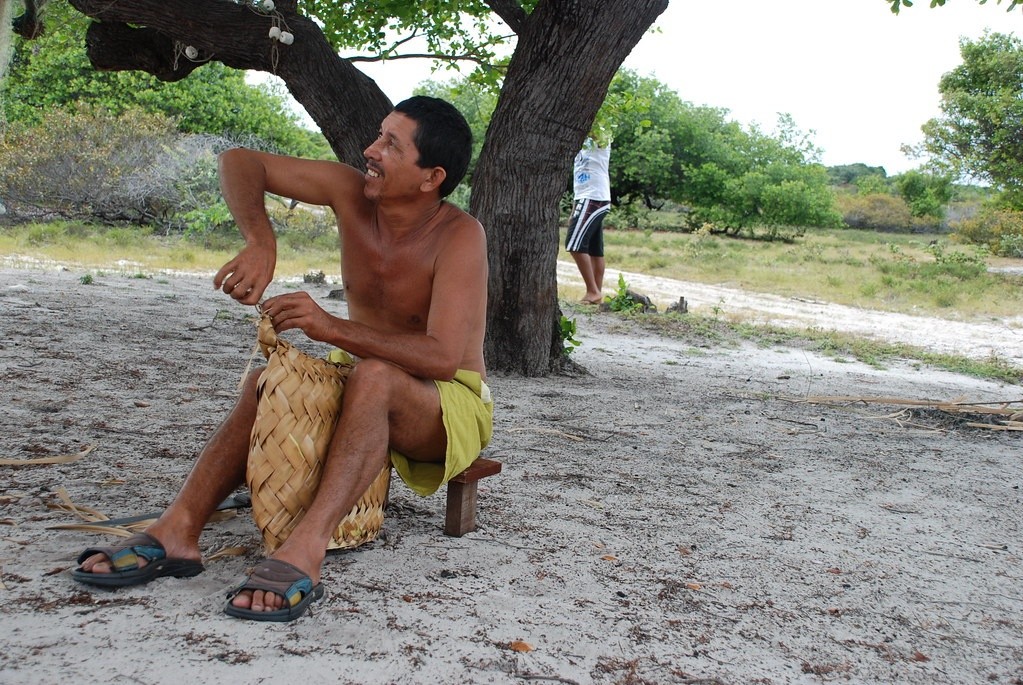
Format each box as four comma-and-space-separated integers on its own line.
445, 458, 502, 536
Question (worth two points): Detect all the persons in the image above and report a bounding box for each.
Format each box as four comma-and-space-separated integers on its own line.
563, 123, 612, 305
68, 95, 493, 621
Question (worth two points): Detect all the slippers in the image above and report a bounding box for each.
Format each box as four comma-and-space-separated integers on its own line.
578, 295, 602, 306
72, 532, 204, 584
225, 559, 324, 621
